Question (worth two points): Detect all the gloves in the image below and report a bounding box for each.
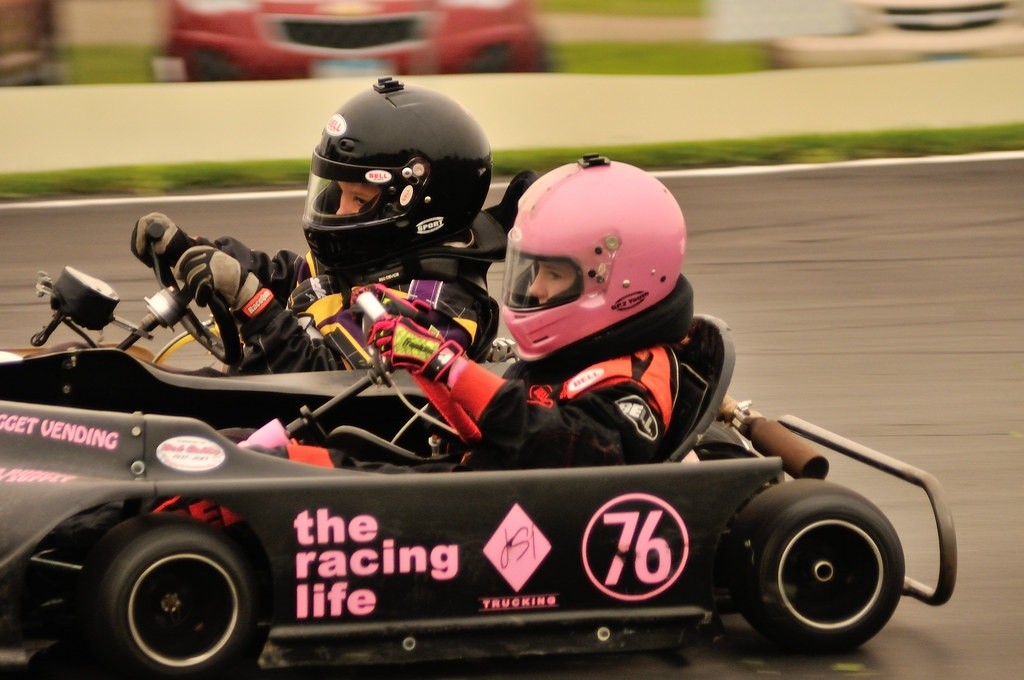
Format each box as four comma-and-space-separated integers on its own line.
367, 313, 463, 382
175, 245, 273, 323
350, 283, 432, 318
131, 211, 202, 268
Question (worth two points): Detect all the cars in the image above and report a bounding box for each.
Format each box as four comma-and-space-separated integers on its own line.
160, 0, 543, 82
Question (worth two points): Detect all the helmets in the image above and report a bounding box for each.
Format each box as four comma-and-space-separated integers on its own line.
501, 151, 685, 363
302, 74, 492, 286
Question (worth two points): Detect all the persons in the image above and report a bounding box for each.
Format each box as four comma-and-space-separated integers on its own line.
128, 77, 499, 383
234, 150, 679, 474
